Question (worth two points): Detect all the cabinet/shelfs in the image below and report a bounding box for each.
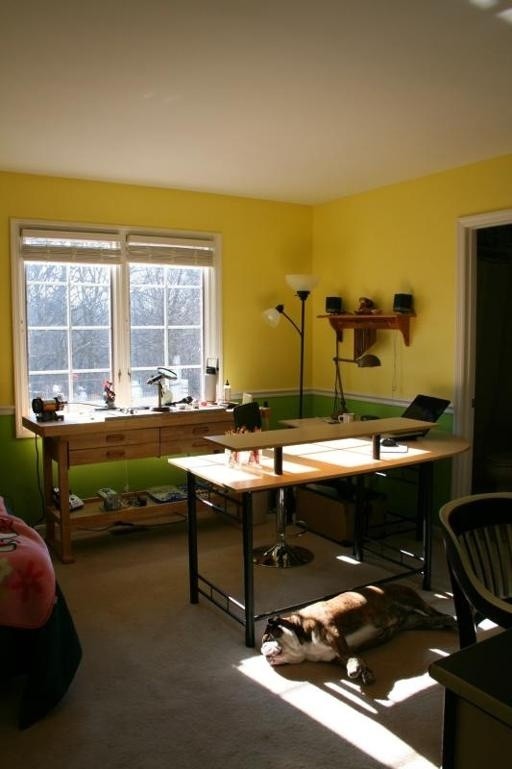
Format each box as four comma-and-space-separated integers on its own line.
17, 402, 273, 565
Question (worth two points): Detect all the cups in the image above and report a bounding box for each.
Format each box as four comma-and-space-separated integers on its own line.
225, 448, 240, 466
338, 413, 354, 423
248, 449, 262, 464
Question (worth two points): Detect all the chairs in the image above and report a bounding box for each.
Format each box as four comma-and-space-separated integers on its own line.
432, 489, 512, 648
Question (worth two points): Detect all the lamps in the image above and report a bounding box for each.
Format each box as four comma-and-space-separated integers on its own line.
329, 354, 382, 421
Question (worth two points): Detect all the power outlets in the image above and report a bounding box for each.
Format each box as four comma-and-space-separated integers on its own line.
260, 271, 320, 421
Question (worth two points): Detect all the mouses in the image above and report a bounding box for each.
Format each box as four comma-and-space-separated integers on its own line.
379, 437, 397, 446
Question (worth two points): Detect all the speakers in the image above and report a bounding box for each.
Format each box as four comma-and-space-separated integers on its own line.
325, 296, 341, 315
393, 293, 413, 314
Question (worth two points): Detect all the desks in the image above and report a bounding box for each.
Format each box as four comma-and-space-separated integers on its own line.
424, 629, 511, 769
165, 416, 472, 652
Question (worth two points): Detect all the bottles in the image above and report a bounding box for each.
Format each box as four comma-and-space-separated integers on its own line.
224, 379, 232, 402
205, 367, 217, 402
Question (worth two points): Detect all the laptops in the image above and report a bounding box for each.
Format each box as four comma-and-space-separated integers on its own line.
369, 393, 451, 439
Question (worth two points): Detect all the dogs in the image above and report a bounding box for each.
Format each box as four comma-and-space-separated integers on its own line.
258, 581, 460, 687
